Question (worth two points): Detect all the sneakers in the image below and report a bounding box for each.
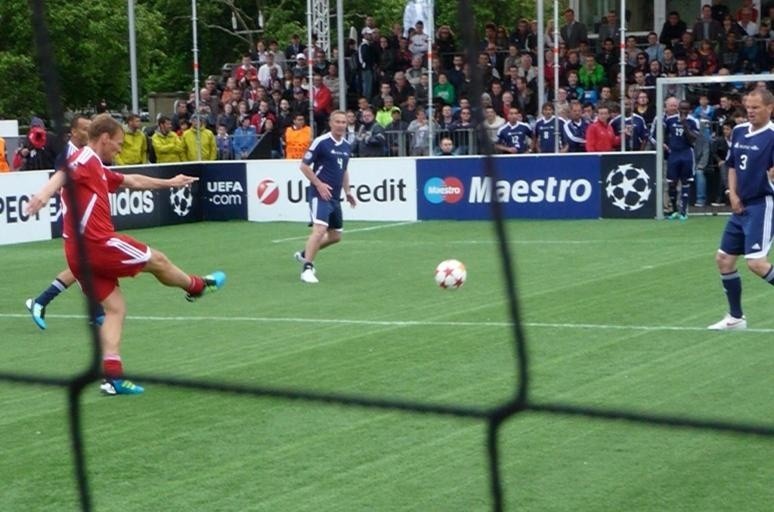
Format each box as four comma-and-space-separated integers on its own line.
295, 252, 316, 273
100, 378, 144, 394
88, 315, 104, 326
301, 269, 319, 283
184, 270, 226, 303
25, 298, 47, 330
707, 313, 747, 331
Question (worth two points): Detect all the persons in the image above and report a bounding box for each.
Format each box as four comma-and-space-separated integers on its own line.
26, 114, 105, 329
347, 0, 773, 220
294, 110, 357, 285
26, 116, 226, 394
1, 33, 343, 173
707, 88, 774, 331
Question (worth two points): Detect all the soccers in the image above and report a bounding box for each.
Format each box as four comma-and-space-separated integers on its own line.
435, 260, 467, 289
